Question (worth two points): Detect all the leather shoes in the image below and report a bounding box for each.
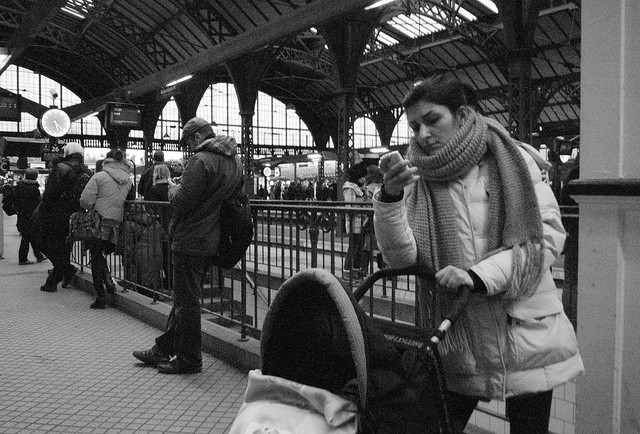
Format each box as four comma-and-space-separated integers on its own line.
62, 264, 78, 288
157, 357, 202, 373
37, 257, 46, 261
133, 344, 171, 366
20, 261, 34, 264
39, 277, 58, 292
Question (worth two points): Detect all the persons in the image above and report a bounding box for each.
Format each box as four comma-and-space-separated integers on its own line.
342, 164, 368, 286
133, 116, 244, 374
256, 178, 338, 201
138, 150, 175, 201
45, 155, 61, 172
147, 164, 174, 203
25, 142, 93, 292
79, 148, 134, 309
371, 73, 585, 434
170, 160, 185, 185
16, 168, 48, 265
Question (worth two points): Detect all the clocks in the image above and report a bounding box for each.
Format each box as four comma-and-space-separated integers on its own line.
41, 109, 71, 138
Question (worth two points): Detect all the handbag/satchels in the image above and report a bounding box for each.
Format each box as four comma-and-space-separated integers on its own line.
68, 207, 101, 241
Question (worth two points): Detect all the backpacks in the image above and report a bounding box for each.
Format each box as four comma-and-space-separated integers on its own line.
58, 160, 94, 207
211, 168, 254, 270
0, 184, 24, 216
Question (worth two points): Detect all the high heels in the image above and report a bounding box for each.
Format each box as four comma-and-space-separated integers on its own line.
90, 292, 106, 309
105, 277, 116, 294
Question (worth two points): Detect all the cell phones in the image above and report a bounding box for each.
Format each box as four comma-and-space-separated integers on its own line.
170, 178, 182, 185
380, 149, 415, 184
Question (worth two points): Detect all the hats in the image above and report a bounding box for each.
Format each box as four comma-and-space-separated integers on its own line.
179, 117, 209, 145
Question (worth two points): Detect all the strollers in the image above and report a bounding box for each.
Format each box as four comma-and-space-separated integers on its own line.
260, 265, 473, 434
